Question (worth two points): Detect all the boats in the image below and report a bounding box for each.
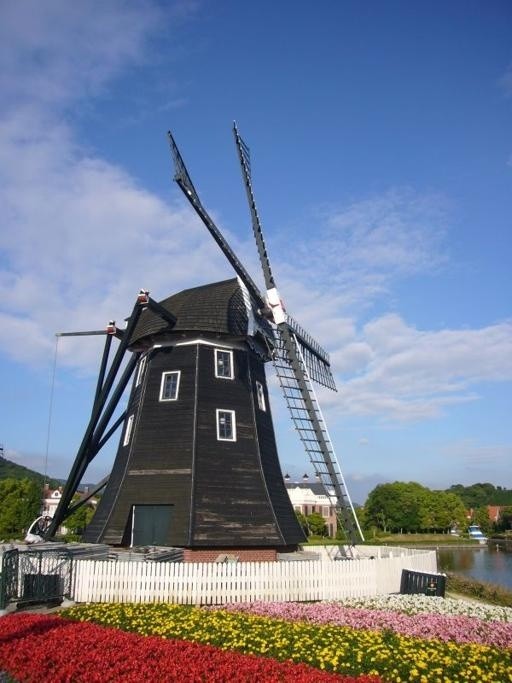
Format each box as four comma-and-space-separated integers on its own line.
449, 522, 489, 545
